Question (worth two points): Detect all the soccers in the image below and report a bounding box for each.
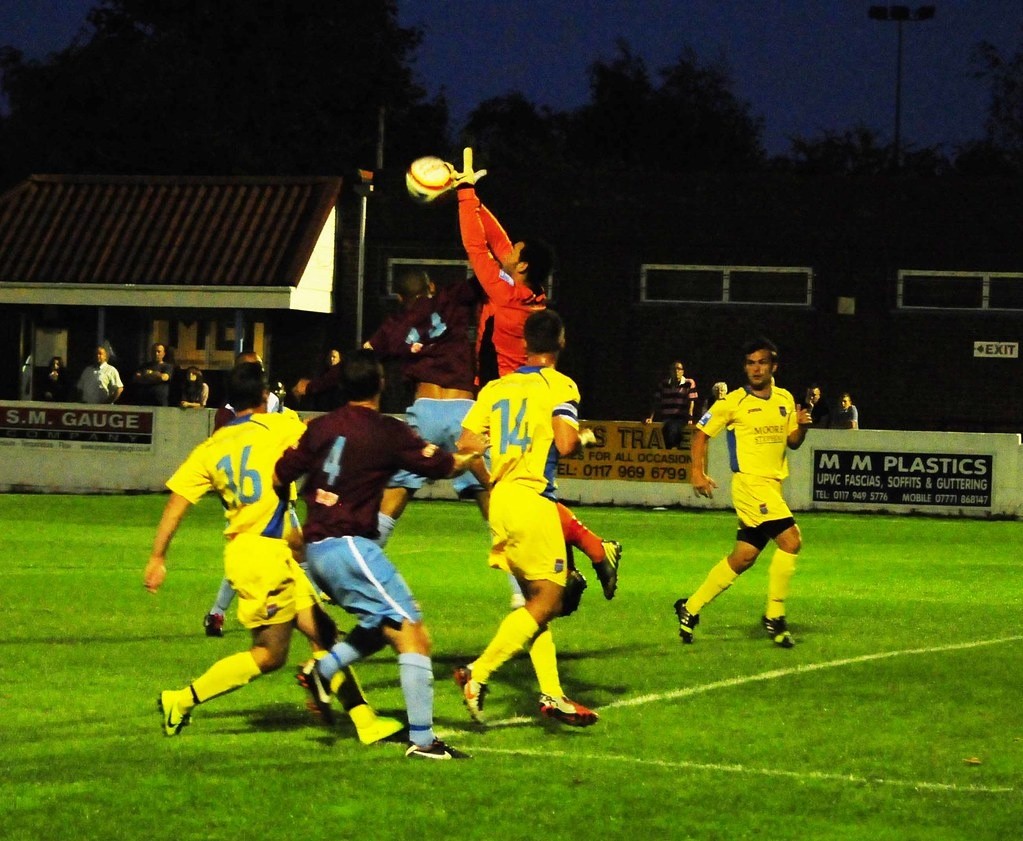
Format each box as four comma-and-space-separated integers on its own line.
405, 156, 453, 201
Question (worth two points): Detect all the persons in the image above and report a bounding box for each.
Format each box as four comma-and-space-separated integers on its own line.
830, 392, 858, 428
179, 366, 210, 408
73, 346, 124, 404
800, 384, 819, 426
703, 381, 728, 417
43, 357, 71, 401
136, 343, 173, 407
143, 147, 625, 759
673, 337, 814, 647
643, 362, 698, 449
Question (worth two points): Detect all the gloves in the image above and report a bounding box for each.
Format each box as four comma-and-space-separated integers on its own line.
448, 146, 486, 187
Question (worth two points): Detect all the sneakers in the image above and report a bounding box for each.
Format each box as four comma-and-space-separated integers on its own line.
203, 611, 226, 637
452, 666, 491, 725
568, 569, 586, 613
354, 713, 403, 746
674, 598, 700, 645
761, 612, 795, 650
158, 688, 192, 737
537, 692, 599, 728
294, 658, 335, 729
406, 741, 472, 760
593, 540, 622, 602
